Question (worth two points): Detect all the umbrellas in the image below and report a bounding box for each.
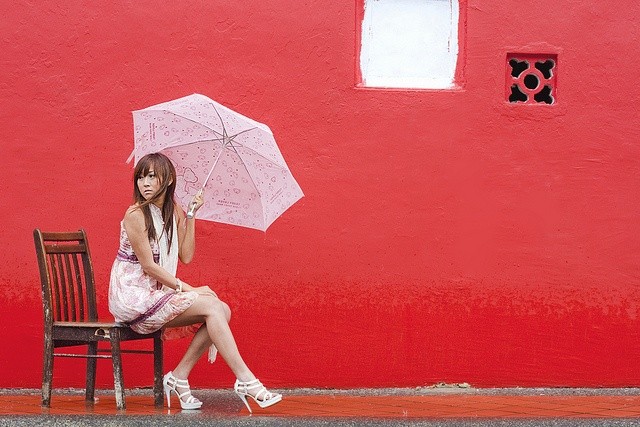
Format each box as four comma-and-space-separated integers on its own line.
131, 93, 305, 233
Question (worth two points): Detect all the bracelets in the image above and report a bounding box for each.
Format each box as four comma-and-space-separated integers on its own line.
175, 277, 182, 293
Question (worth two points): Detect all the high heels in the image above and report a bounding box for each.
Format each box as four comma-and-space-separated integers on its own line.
234, 378, 282, 414
163, 370, 203, 410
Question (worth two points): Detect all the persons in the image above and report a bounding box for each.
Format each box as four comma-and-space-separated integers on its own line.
108, 152, 282, 413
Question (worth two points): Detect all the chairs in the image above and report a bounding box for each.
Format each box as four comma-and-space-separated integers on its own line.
33, 226, 164, 411
